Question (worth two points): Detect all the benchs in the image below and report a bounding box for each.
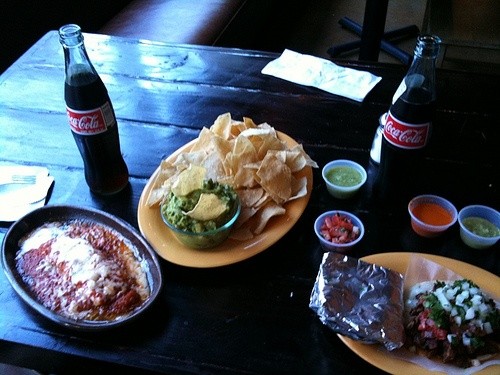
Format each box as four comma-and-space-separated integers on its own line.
97, 0, 245, 45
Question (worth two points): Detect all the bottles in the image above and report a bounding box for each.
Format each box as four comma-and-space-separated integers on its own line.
369, 112, 387, 164
377, 32, 443, 186
59, 24, 130, 197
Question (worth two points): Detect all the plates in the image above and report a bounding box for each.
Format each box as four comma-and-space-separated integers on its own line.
0, 204, 163, 332
136, 127, 312, 268
335, 252, 500, 375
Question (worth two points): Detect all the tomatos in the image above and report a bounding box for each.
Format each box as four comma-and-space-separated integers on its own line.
319, 215, 356, 243
418, 310, 459, 340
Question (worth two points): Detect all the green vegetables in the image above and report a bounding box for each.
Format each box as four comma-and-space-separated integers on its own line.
161, 178, 239, 248
421, 278, 497, 351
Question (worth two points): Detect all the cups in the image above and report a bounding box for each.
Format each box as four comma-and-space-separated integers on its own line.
457, 205, 500, 249
322, 160, 367, 199
407, 194, 457, 238
314, 210, 364, 254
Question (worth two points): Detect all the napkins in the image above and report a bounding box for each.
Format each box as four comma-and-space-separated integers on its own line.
262, 48, 382, 102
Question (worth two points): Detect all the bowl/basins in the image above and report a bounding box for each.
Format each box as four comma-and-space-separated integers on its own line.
160, 181, 241, 250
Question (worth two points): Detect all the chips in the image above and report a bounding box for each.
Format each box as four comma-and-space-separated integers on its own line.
146, 114, 319, 240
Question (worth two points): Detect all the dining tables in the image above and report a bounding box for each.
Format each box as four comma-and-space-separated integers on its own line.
0, 30, 500, 375
